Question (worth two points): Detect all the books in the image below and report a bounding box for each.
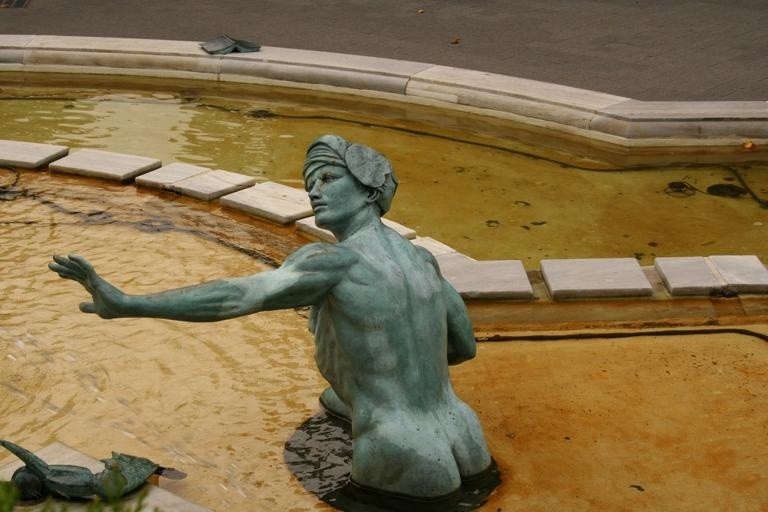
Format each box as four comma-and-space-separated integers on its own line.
200, 33, 261, 57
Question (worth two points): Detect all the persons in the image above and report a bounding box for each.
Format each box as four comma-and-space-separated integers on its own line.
48, 133, 493, 499
